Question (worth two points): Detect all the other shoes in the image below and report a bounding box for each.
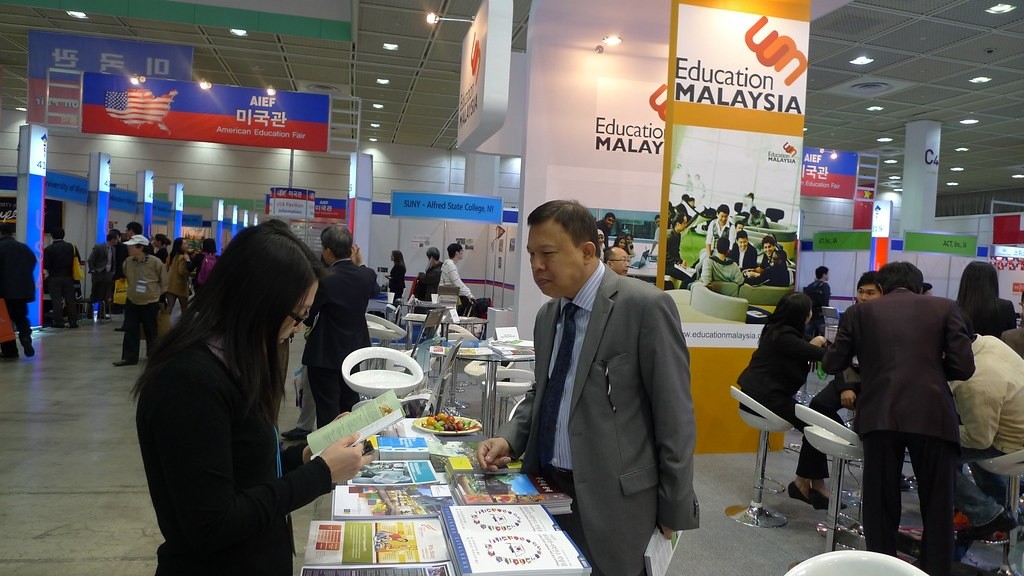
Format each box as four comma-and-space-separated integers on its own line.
901, 474, 911, 491
70, 324, 79, 328
112, 360, 137, 366
50, 324, 65, 328
0, 352, 19, 359
87, 313, 110, 319
115, 327, 125, 332
21, 338, 35, 357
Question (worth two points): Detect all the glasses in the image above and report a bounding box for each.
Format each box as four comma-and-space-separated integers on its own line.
127, 228, 132, 231
289, 309, 309, 328
608, 258, 630, 264
461, 250, 464, 252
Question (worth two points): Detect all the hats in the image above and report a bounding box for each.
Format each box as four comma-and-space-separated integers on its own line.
123, 235, 149, 247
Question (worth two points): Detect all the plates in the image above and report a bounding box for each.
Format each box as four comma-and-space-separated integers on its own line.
412, 416, 482, 435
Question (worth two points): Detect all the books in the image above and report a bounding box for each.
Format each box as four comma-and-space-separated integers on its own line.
299, 438, 593, 576
307, 389, 406, 461
644, 525, 682, 576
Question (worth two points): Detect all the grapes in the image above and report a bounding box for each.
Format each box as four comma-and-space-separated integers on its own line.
427, 416, 463, 431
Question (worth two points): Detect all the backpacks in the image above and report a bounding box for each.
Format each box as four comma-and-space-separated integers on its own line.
803, 281, 829, 315
198, 252, 217, 284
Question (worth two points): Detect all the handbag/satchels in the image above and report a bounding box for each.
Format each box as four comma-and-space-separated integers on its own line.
139, 310, 172, 341
73, 243, 83, 280
0, 299, 16, 343
113, 278, 128, 304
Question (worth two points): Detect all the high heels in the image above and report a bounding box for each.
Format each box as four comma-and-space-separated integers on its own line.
788, 481, 813, 505
813, 489, 848, 509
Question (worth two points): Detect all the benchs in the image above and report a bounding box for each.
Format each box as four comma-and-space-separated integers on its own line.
664, 223, 797, 324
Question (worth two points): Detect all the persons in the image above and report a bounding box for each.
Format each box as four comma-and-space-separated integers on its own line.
129, 225, 373, 576
737, 291, 847, 509
593, 193, 790, 287
477, 200, 702, 576
806, 260, 1024, 540
0, 220, 475, 442
822, 262, 976, 576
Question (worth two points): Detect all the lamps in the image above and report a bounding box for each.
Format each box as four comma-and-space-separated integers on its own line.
595, 34, 622, 52
426, 12, 474, 23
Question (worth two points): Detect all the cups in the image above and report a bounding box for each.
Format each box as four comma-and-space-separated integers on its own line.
388, 292, 395, 303
431, 294, 438, 304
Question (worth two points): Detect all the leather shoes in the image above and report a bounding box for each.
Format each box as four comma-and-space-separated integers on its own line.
281, 427, 312, 439
956, 509, 1017, 541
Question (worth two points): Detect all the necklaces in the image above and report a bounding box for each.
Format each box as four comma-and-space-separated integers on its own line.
208, 342, 225, 351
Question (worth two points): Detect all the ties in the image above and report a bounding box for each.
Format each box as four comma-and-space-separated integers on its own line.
535, 303, 580, 476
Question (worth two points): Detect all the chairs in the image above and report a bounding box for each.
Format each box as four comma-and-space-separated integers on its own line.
821, 305, 840, 341
77, 281, 107, 324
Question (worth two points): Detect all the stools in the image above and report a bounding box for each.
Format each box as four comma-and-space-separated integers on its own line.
783, 550, 929, 576
976, 450, 1024, 576
795, 404, 863, 553
341, 313, 535, 430
725, 386, 794, 529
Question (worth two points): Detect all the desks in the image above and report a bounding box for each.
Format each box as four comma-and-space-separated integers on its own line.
375, 301, 450, 350
402, 316, 488, 374
427, 340, 535, 439
